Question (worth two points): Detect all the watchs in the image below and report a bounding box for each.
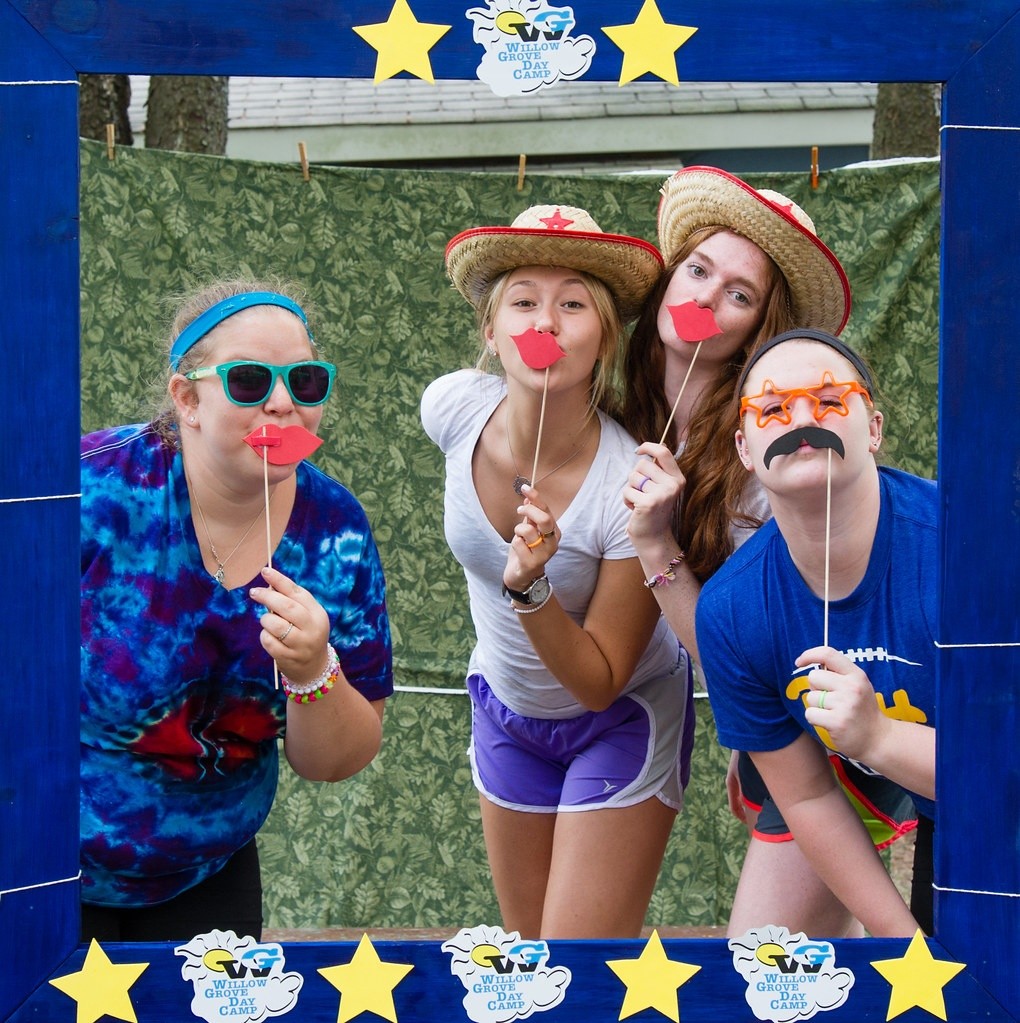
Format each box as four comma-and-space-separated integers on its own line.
502, 573, 551, 606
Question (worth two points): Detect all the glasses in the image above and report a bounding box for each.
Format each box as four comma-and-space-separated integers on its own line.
184, 359, 336, 405
739, 370, 872, 428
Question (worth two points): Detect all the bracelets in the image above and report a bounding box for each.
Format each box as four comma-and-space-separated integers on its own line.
510, 582, 553, 614
644, 551, 684, 589
280, 643, 340, 704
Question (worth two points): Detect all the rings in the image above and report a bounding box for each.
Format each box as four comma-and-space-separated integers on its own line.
819, 690, 827, 712
639, 478, 651, 491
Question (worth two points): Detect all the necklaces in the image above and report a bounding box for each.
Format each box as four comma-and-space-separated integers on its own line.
183, 455, 278, 585
504, 405, 599, 497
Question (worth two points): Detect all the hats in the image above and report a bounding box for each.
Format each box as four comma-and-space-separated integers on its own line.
655, 165, 851, 338
442, 204, 662, 326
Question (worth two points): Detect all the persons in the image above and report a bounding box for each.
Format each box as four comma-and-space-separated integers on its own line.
683, 326, 938, 939
78, 281, 395, 950
421, 204, 698, 941
600, 168, 891, 882
